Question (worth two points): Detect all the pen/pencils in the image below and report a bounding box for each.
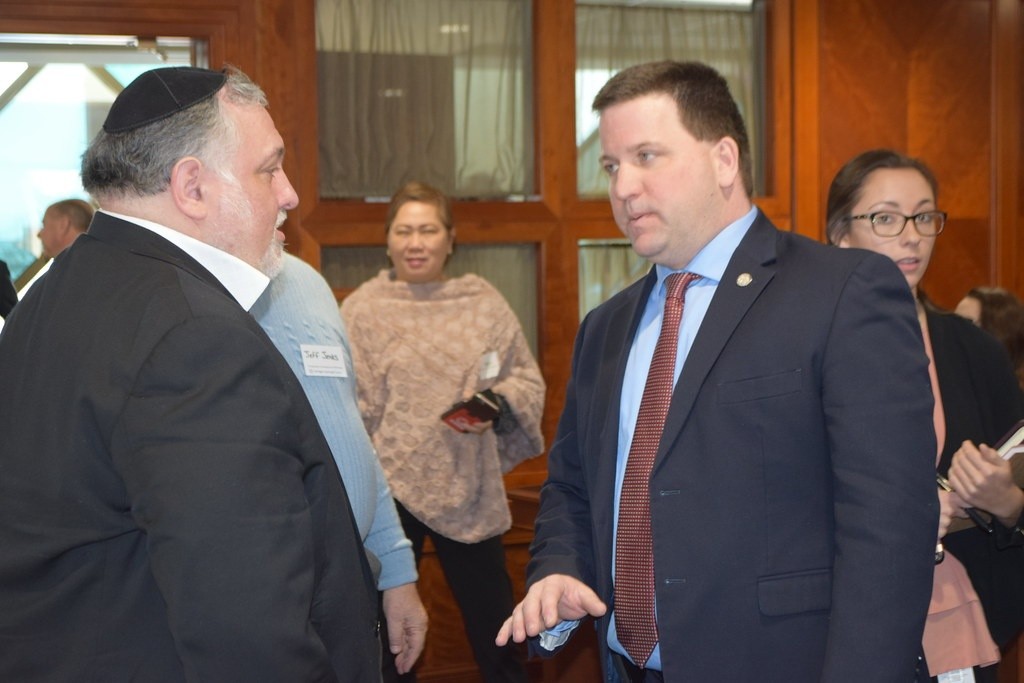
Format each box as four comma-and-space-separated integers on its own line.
936, 471, 993, 533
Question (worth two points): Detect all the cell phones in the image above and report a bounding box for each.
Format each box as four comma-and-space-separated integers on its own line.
439, 390, 503, 433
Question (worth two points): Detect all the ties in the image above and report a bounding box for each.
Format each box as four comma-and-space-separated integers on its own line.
614, 271, 706, 669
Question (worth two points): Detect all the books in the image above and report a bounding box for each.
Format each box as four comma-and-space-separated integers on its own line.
945, 443, 1024, 534
991, 416, 1024, 458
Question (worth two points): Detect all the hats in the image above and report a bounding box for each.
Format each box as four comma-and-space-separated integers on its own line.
101, 67, 227, 133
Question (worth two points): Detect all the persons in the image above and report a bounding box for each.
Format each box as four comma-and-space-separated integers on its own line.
243, 247, 430, 682
338, 181, 546, 682
826, 148, 1024, 683
38, 198, 96, 258
954, 287, 1024, 372
493, 59, 940, 681
0, 259, 20, 321
0, 61, 400, 682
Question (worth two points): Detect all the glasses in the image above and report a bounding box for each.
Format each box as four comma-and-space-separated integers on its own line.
849, 211, 947, 237
